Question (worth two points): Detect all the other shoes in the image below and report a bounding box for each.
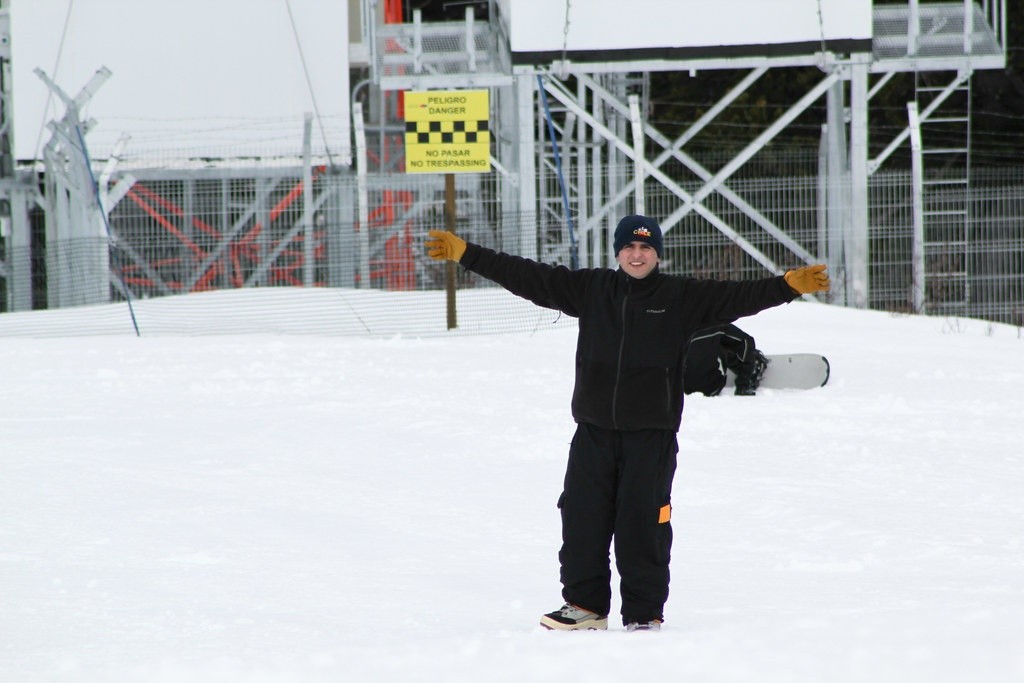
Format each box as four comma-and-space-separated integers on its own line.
705, 375, 727, 396
734, 349, 768, 395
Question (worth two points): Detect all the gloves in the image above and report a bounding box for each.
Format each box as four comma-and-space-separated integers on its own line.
783, 265, 830, 295
424, 230, 466, 263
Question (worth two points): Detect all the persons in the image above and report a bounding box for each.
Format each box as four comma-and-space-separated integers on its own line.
424, 213, 831, 635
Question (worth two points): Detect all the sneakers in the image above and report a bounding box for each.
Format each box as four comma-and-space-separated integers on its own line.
627, 620, 659, 631
540, 602, 608, 630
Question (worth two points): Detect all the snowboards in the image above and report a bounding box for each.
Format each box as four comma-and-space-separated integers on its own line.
725, 351, 832, 392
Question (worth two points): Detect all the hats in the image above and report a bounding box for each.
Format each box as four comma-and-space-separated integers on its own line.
613, 216, 662, 258
